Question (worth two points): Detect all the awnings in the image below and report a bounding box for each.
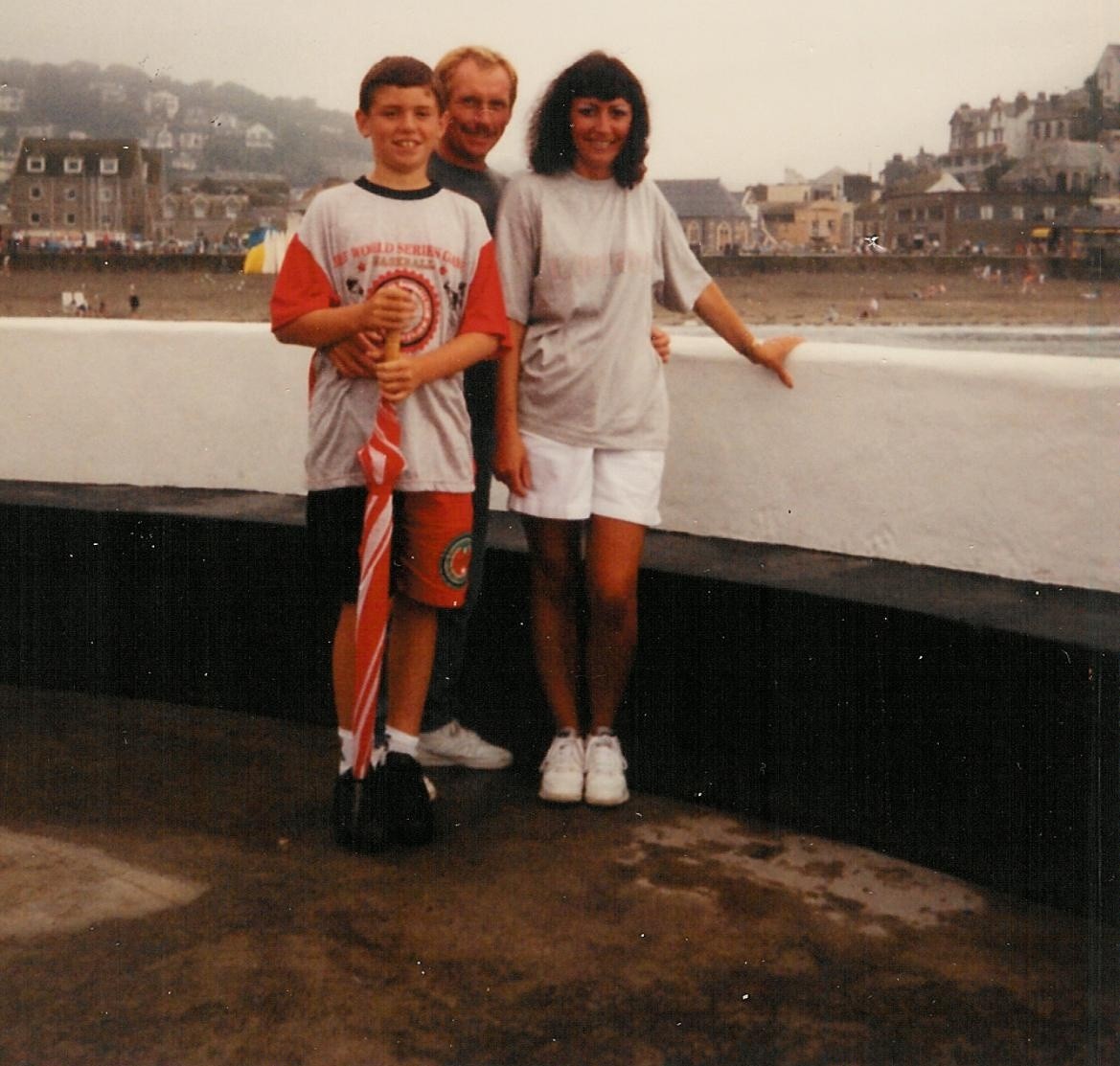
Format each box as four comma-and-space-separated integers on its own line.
1033, 229, 1049, 238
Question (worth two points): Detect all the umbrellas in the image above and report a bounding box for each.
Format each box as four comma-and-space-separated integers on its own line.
347, 284, 410, 844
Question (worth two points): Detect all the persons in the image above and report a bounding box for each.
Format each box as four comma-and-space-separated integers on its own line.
74, 289, 139, 317
270, 55, 514, 854
322, 46, 671, 768
8, 230, 209, 253
497, 51, 802, 806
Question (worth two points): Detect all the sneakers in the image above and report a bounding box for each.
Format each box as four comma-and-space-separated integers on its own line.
582, 726, 629, 805
538, 729, 586, 802
333, 764, 398, 842
374, 750, 433, 844
414, 719, 512, 769
